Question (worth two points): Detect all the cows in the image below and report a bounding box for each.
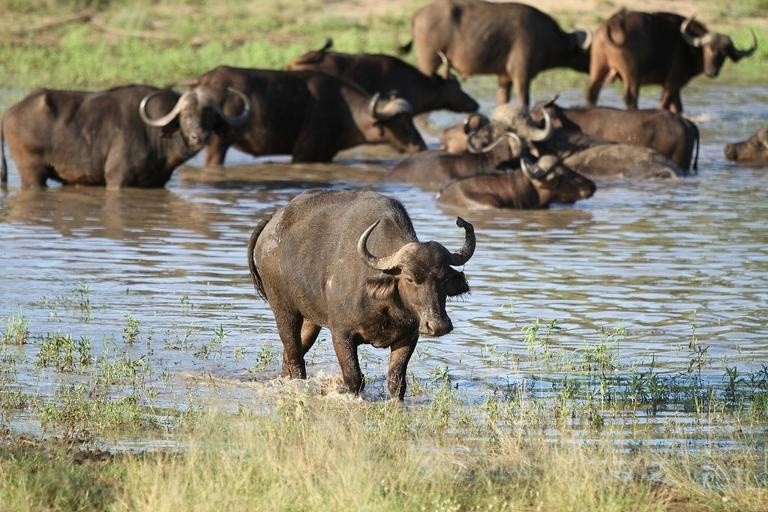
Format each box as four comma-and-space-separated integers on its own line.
384, 94, 700, 211
723, 127, 768, 168
585, 7, 758, 114
247, 188, 476, 402
0, 84, 252, 194
396, 0, 590, 107
186, 65, 427, 168
293, 39, 480, 117
